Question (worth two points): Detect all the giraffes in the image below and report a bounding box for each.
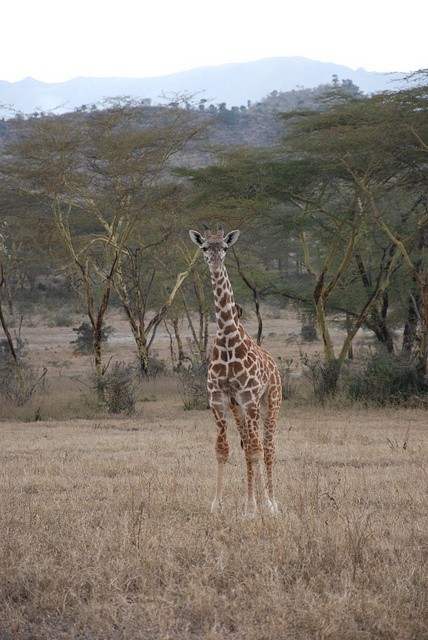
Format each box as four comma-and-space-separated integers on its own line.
188, 224, 282, 519
